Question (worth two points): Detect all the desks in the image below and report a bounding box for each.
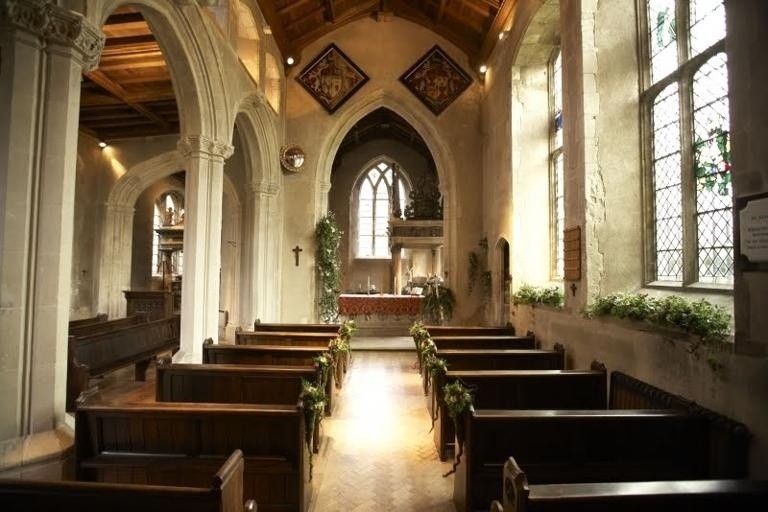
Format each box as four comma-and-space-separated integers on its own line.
122, 290, 181, 320
337, 292, 428, 329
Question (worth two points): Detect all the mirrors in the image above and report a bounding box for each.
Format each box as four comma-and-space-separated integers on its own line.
280, 143, 309, 172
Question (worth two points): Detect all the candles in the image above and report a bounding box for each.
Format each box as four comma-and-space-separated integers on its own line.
393, 276, 396, 296
367, 275, 370, 296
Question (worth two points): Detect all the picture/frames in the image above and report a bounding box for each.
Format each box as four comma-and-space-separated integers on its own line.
398, 44, 475, 118
734, 191, 768, 274
294, 43, 370, 116
